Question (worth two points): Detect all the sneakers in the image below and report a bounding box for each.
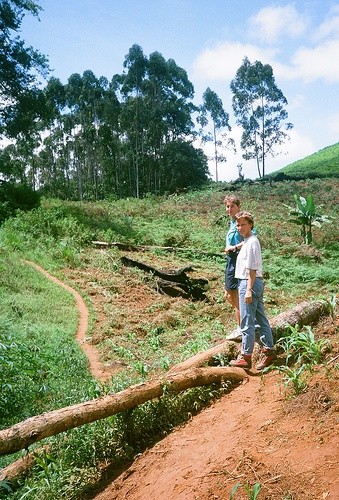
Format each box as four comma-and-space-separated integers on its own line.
225, 329, 242, 340
230, 354, 252, 370
256, 349, 277, 370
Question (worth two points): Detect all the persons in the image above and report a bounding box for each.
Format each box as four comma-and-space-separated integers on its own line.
225, 194, 256, 332
230, 210, 276, 369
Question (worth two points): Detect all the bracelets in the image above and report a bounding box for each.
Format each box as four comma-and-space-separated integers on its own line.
233, 245, 237, 253
